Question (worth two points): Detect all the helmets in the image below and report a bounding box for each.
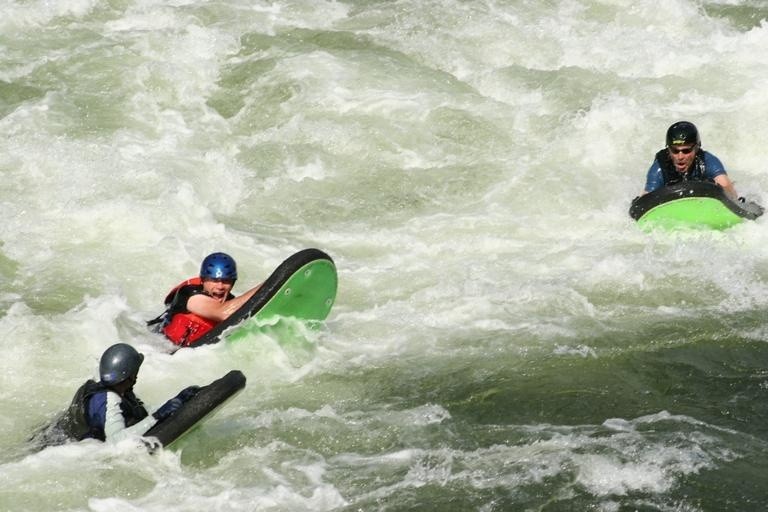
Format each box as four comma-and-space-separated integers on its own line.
100, 343, 144, 387
666, 121, 700, 147
200, 252, 237, 280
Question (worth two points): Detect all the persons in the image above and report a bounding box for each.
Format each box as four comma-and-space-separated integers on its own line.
152, 251, 262, 347
37, 342, 201, 447
638, 120, 738, 201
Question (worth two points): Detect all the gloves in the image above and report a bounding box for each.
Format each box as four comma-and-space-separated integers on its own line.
175, 386, 198, 403
151, 398, 184, 425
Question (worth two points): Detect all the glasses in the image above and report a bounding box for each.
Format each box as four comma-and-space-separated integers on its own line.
668, 143, 696, 154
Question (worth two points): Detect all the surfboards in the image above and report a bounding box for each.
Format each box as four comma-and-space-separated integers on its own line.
190, 248, 340, 350
628, 181, 763, 234
141, 370, 245, 454
